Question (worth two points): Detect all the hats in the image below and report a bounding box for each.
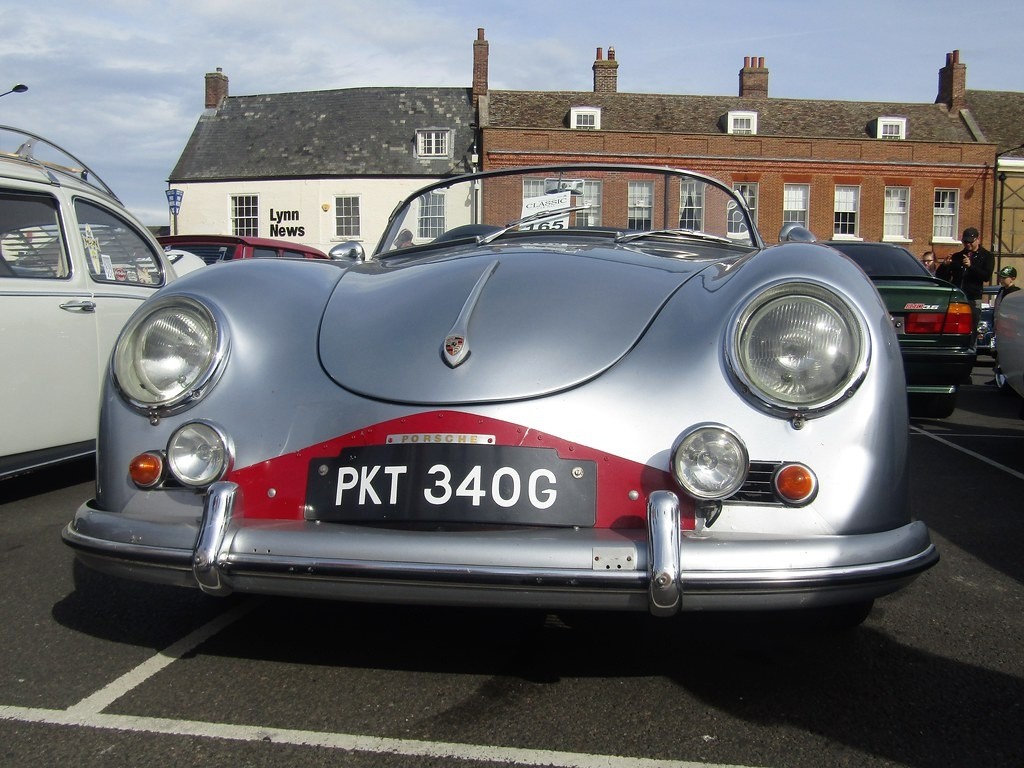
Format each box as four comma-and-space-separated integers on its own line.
394, 229, 413, 245
962, 227, 979, 241
994, 266, 1017, 279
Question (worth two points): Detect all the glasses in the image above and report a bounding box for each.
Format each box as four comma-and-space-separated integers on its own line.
921, 260, 933, 262
964, 241, 973, 243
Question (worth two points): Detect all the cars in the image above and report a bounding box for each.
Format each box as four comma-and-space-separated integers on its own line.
976, 286, 1003, 358
60, 163, 941, 626
995, 290, 1024, 398
820, 240, 978, 418
0, 124, 179, 481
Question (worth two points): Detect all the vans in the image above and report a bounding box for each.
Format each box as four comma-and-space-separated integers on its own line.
129, 235, 331, 286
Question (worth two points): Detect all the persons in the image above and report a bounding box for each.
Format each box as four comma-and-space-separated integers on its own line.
921, 252, 936, 277
935, 227, 995, 385
393, 228, 416, 250
991, 265, 1021, 335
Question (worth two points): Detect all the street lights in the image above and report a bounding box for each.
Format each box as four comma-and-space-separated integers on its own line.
994, 173, 1008, 285
0, 84, 28, 97
469, 123, 478, 224
988, 144, 1024, 304
165, 189, 184, 235
